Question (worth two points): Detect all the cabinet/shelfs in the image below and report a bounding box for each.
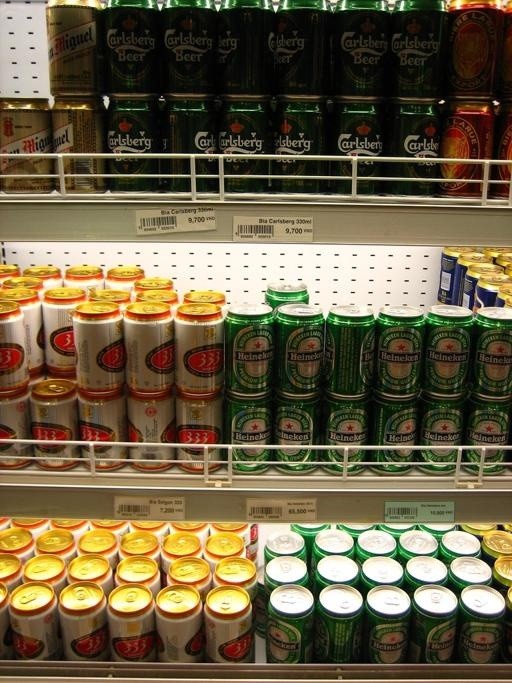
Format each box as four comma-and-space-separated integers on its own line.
0, 152, 512, 683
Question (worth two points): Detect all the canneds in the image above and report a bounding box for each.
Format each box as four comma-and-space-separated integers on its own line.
0, 245, 512, 477
0, 0, 512, 200
0, 516, 512, 665
